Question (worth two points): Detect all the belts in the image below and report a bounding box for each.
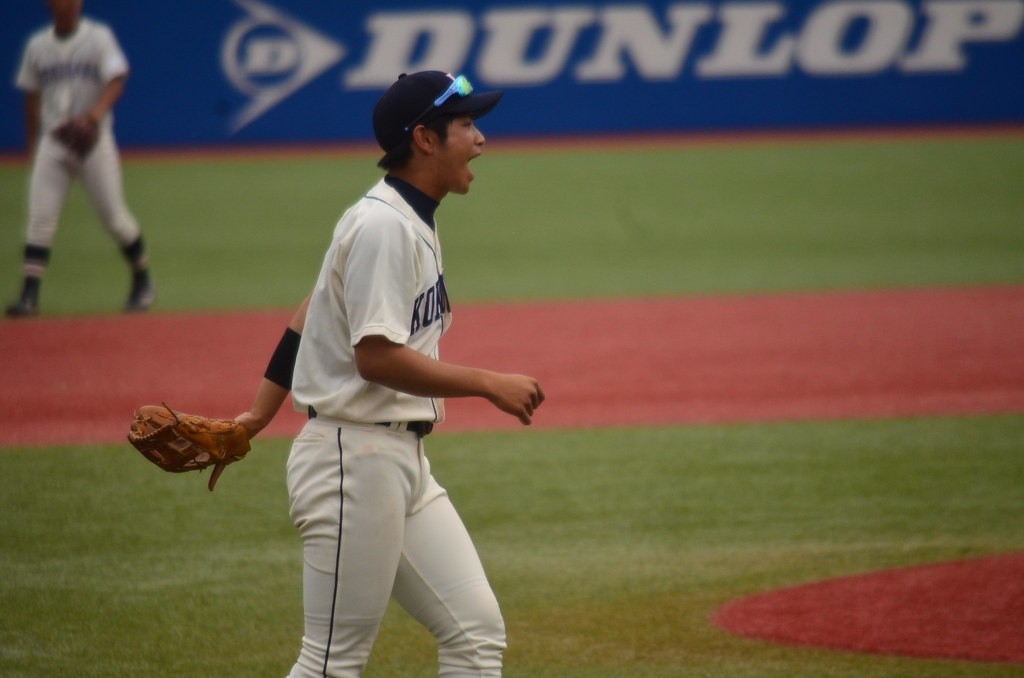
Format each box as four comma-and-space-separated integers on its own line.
379, 421, 435, 439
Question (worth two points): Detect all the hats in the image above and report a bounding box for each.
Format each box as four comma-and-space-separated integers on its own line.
372, 70, 504, 152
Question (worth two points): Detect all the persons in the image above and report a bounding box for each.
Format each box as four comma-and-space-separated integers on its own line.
7, 0, 154, 315
127, 70, 544, 676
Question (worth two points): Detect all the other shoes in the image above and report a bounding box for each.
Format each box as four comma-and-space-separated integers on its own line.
125, 271, 155, 310
6, 292, 40, 316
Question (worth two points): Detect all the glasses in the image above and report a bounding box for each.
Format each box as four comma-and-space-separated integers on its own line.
404, 76, 474, 130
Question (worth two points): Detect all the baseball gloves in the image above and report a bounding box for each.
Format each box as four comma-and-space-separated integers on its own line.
50, 115, 100, 160
128, 405, 252, 474
